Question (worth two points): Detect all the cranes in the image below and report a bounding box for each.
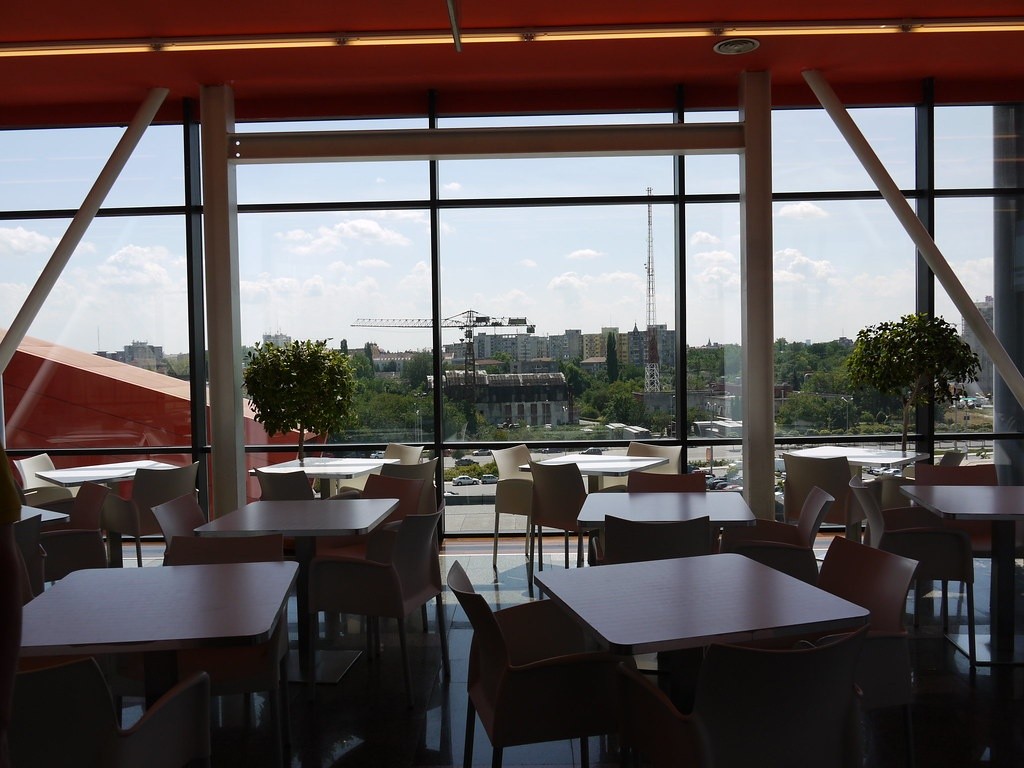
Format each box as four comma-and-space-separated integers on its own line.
350, 308, 536, 434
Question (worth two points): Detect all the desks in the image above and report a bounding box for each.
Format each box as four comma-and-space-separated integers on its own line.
17, 560, 299, 712
249, 457, 401, 499
575, 491, 758, 531
532, 553, 874, 714
35, 460, 181, 568
898, 485, 1024, 668
791, 446, 930, 544
519, 454, 670, 566
193, 497, 402, 685
20, 505, 70, 527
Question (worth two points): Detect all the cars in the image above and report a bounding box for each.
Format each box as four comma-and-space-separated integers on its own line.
472, 448, 492, 456
481, 474, 497, 484
581, 447, 602, 455
370, 451, 385, 458
862, 465, 902, 477
453, 475, 480, 486
541, 447, 562, 453
688, 463, 744, 490
455, 458, 479, 466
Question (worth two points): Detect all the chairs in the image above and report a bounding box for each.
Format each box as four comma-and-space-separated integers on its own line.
12, 439, 1024, 768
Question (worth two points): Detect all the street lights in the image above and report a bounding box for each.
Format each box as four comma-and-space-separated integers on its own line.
842, 396, 853, 434
707, 401, 716, 431
976, 392, 991, 449
670, 395, 675, 437
